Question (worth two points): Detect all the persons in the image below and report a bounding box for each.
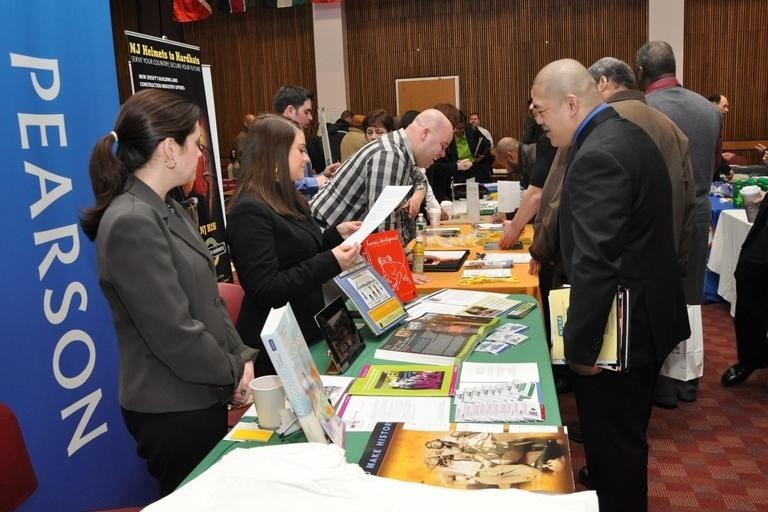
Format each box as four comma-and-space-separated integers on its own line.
229, 86, 560, 305
707, 95, 735, 183
76, 87, 258, 495
529, 58, 692, 512
527, 57, 696, 485
224, 114, 365, 379
720, 143, 767, 388
635, 43, 721, 411
423, 438, 569, 496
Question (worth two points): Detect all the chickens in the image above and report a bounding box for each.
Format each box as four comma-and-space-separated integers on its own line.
314, 296, 365, 374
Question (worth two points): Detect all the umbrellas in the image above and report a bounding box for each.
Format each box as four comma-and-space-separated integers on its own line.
722, 360, 767, 386
579, 466, 590, 486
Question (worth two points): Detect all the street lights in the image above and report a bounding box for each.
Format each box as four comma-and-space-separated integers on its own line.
660, 304, 704, 382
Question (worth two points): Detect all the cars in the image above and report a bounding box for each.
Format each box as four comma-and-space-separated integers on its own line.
428, 208, 441, 230
739, 186, 761, 223
249, 374, 287, 430
441, 200, 453, 221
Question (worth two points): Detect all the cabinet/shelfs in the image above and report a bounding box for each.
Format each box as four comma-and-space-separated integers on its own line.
732, 174, 768, 209
415, 213, 427, 239
411, 237, 425, 274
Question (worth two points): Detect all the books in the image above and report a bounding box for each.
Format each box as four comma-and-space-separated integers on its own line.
362, 229, 417, 304
374, 312, 501, 368
260, 302, 334, 444
334, 263, 408, 336
347, 365, 459, 397
457, 296, 538, 319
547, 283, 630, 371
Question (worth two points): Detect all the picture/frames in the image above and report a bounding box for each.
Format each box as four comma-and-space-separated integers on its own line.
704, 160, 768, 318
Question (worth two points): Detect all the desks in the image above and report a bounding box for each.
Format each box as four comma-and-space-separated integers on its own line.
171, 294, 566, 510
704, 160, 768, 318
402, 193, 545, 310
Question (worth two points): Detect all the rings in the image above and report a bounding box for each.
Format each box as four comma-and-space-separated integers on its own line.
242, 389, 246, 396
